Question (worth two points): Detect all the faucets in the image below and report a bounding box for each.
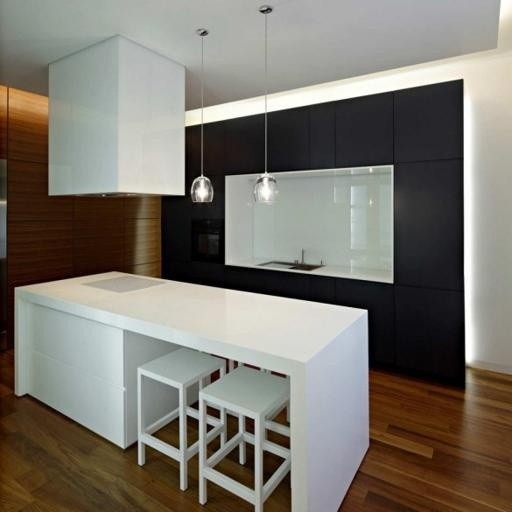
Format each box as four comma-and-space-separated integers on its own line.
301, 249, 305, 263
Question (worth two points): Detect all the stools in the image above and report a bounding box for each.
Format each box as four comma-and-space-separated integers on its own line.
137, 348, 227, 492
198, 366, 290, 512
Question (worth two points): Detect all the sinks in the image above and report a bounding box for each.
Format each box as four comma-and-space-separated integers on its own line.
257, 261, 297, 270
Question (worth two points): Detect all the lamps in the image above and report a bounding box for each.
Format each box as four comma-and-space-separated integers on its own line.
253, 6, 278, 203
190, 27, 215, 204
